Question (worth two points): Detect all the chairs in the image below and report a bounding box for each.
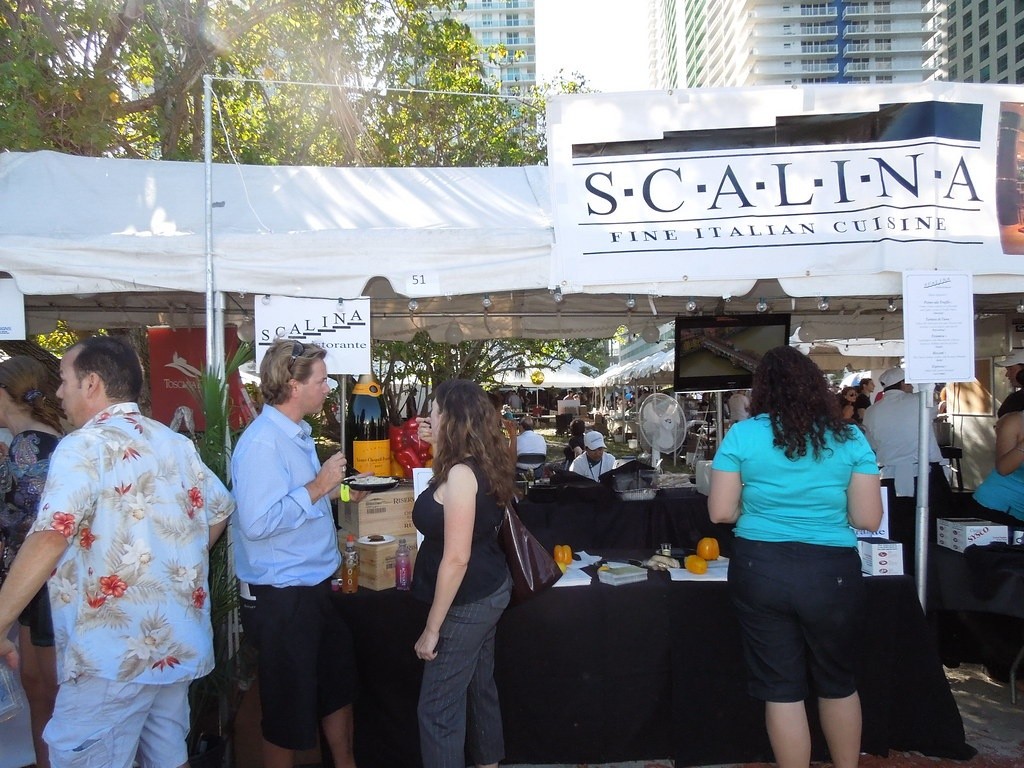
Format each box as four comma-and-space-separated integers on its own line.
515, 452, 546, 481
564, 445, 586, 472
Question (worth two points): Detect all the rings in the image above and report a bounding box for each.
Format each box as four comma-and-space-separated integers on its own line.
343, 465, 347, 473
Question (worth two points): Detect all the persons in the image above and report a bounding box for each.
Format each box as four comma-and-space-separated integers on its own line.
0, 337, 234, 768
487, 386, 750, 483
407, 388, 417, 421
0, 355, 68, 768
838, 348, 1024, 530
231, 338, 356, 768
710, 346, 882, 768
411, 379, 513, 768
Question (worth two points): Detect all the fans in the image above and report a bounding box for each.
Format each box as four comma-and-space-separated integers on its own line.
639, 393, 687, 474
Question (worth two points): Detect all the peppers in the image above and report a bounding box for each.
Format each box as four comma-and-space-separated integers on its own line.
553, 544, 572, 572
389, 416, 436, 479
697, 537, 720, 560
685, 554, 707, 574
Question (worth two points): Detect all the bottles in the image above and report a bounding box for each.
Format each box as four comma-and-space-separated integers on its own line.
344, 369, 391, 476
395, 539, 412, 590
527, 469, 536, 488
660, 543, 671, 556
342, 535, 359, 593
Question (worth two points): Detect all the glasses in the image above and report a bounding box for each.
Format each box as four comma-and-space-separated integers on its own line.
849, 392, 856, 397
288, 340, 304, 370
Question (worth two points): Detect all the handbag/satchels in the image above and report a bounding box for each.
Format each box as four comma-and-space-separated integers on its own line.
499, 501, 563, 604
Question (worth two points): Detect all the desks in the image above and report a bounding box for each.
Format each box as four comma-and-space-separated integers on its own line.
513, 412, 556, 428
686, 431, 716, 458
606, 418, 637, 438
513, 486, 737, 545
326, 544, 978, 768
928, 542, 1024, 704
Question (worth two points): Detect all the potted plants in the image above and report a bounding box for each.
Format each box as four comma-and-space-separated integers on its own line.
185, 342, 258, 768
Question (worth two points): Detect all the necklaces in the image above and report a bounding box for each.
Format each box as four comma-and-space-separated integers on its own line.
586, 455, 602, 482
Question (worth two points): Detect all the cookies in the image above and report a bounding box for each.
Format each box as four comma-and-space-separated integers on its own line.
367, 535, 384, 540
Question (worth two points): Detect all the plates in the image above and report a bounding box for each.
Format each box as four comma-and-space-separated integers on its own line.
357, 535, 395, 544
340, 476, 404, 492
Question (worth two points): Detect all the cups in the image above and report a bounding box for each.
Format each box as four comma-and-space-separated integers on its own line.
0, 659, 24, 722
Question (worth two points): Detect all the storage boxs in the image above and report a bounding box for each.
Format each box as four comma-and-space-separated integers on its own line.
936, 518, 1009, 553
685, 438, 714, 495
612, 435, 622, 442
549, 405, 606, 424
856, 537, 905, 576
333, 483, 417, 591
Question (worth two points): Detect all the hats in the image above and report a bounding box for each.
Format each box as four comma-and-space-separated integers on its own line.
993, 349, 1024, 367
879, 368, 905, 388
584, 431, 607, 450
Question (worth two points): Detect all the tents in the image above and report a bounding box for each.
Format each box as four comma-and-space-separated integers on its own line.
1, 153, 1024, 443
